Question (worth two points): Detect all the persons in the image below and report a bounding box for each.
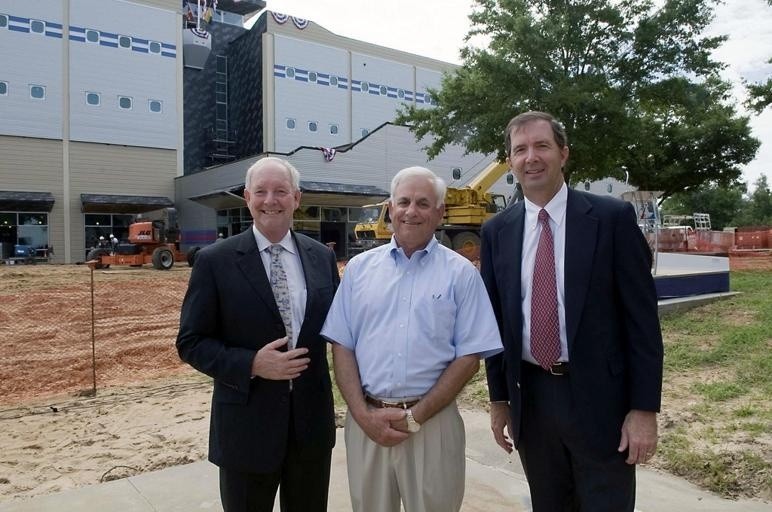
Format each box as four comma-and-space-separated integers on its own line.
109, 233, 118, 248
318, 166, 505, 512
216, 233, 223, 242
99, 235, 108, 246
480, 112, 664, 512
175, 156, 340, 512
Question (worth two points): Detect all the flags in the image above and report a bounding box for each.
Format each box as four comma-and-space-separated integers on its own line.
187, 0, 212, 24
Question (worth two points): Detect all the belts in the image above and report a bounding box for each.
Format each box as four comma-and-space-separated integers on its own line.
550, 362, 563, 376
366, 395, 419, 410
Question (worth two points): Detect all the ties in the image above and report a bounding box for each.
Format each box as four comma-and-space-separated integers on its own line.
530, 209, 561, 370
267, 244, 293, 351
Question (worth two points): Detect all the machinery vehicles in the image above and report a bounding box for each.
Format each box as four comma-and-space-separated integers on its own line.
86, 219, 200, 269
350, 149, 514, 256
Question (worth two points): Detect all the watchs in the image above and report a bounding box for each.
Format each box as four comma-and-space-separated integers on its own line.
405, 409, 420, 433
